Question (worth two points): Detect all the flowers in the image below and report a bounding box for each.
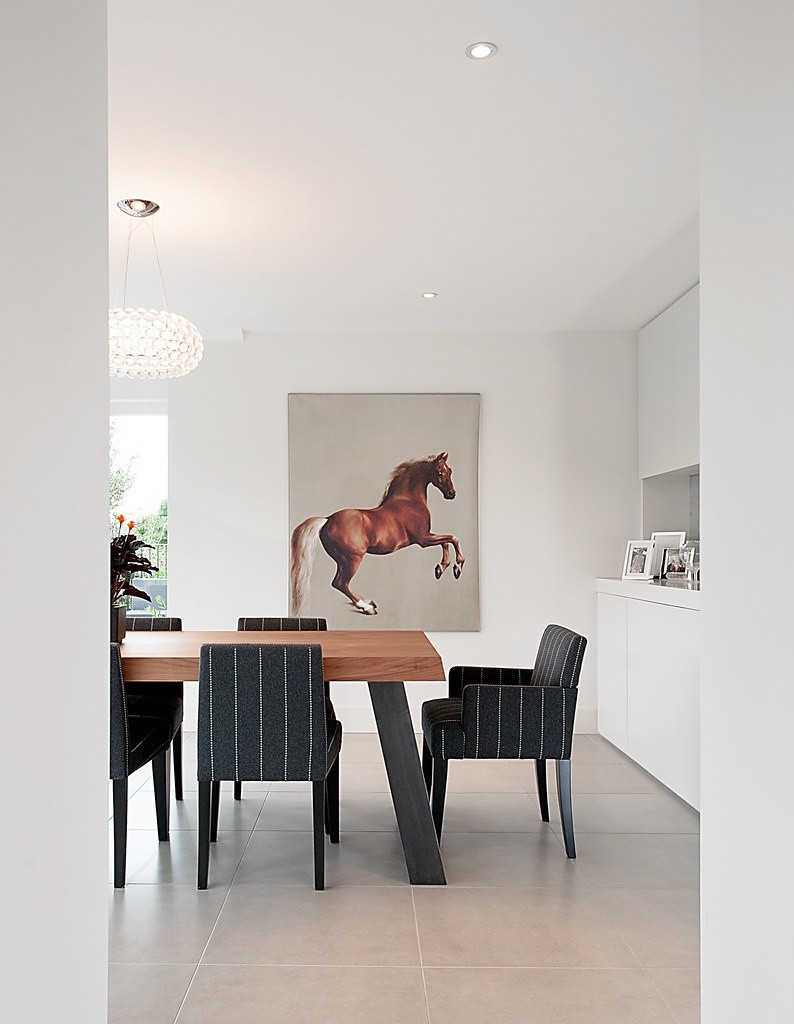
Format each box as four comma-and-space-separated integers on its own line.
110, 515, 160, 607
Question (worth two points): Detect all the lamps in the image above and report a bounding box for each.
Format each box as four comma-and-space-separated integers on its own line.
109, 198, 205, 380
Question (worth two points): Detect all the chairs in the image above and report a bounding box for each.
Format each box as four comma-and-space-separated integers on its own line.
236, 616, 337, 835
196, 643, 343, 892
421, 624, 587, 859
126, 617, 183, 830
110, 643, 171, 888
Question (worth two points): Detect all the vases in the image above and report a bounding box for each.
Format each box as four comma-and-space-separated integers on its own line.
110, 604, 126, 645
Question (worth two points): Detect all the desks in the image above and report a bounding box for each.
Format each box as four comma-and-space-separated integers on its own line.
110, 631, 448, 886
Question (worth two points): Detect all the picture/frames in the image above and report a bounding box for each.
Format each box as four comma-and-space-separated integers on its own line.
622, 540, 656, 580
650, 532, 685, 576
659, 548, 695, 582
